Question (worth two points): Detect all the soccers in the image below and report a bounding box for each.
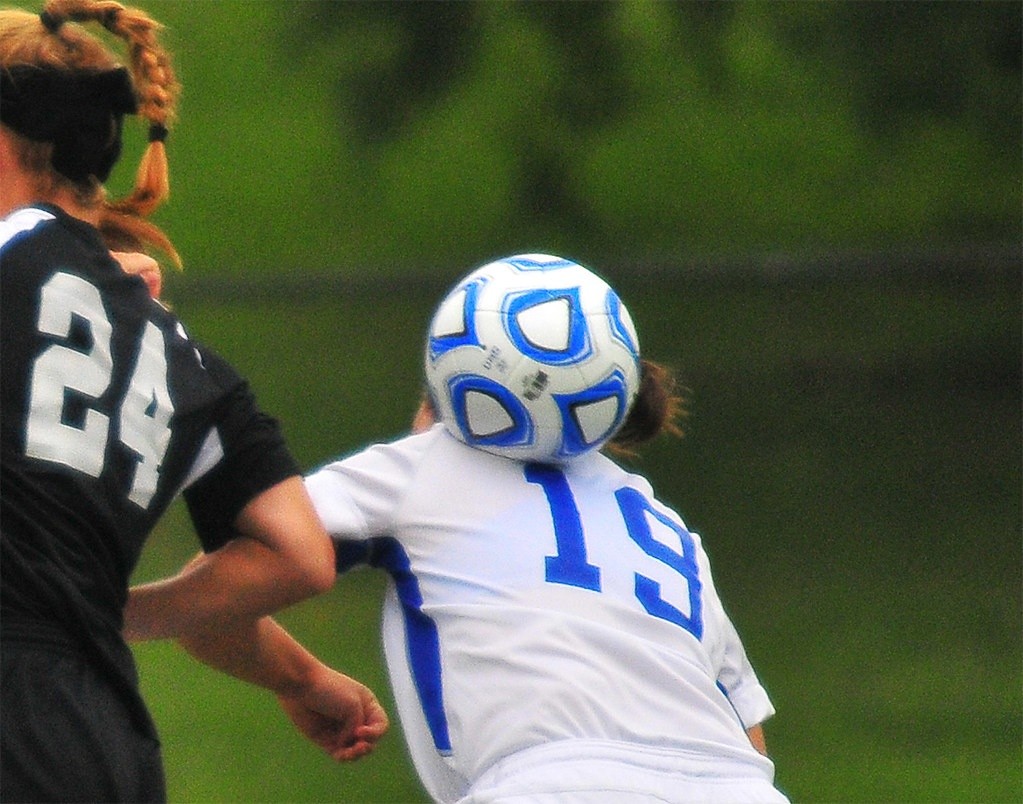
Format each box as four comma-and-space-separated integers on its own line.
423, 250, 644, 463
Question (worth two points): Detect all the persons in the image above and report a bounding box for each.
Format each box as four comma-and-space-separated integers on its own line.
177, 361, 791, 804
0, 1, 335, 804
